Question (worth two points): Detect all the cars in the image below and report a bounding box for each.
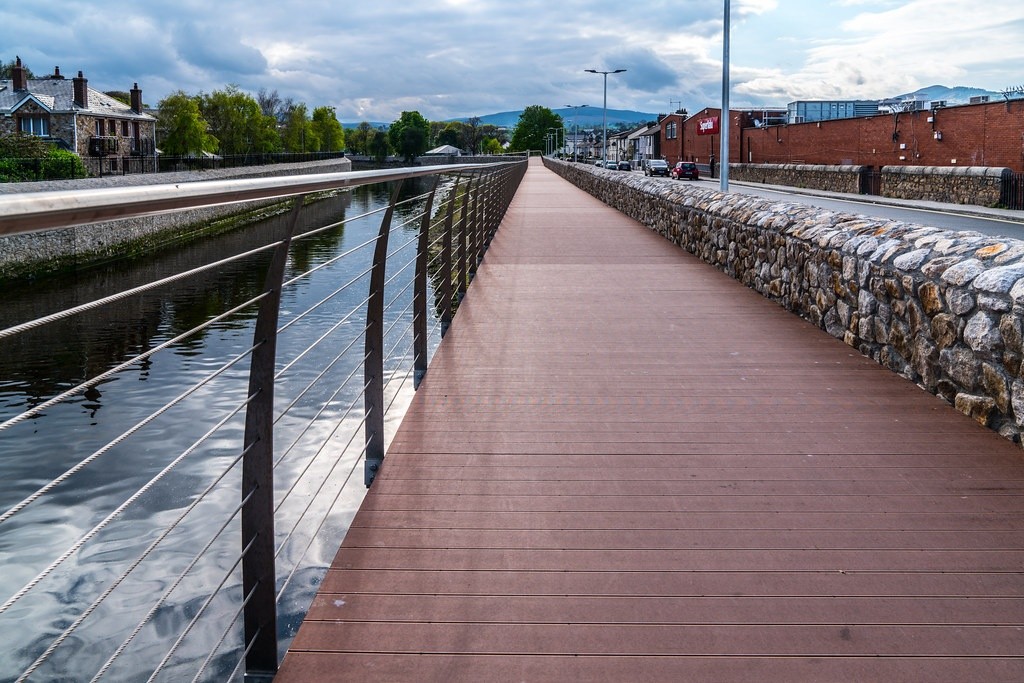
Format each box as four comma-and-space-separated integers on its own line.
618, 161, 631, 171
567, 157, 572, 161
594, 160, 603, 167
645, 159, 670, 177
560, 155, 564, 160
605, 160, 617, 170
671, 161, 699, 180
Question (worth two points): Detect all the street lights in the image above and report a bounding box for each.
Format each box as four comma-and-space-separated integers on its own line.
555, 120, 572, 159
563, 104, 589, 162
542, 127, 564, 159
584, 69, 627, 169
